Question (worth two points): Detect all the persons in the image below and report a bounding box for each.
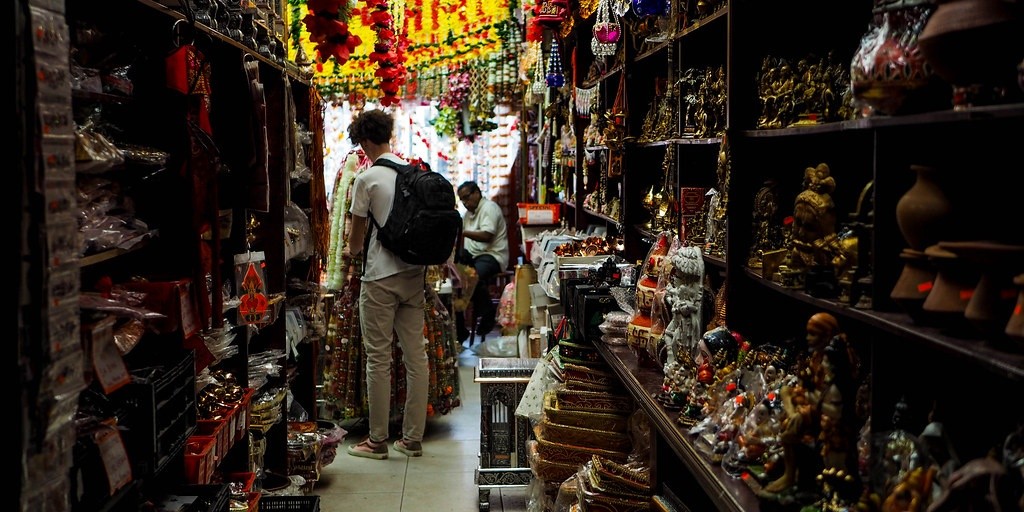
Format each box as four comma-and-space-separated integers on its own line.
458, 181, 508, 335
341, 109, 429, 459
637, 0, 1024, 512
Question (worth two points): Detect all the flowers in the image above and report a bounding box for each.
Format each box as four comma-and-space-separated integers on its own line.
285, 0, 521, 140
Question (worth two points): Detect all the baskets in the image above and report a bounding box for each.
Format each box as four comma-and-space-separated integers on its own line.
527, 338, 650, 512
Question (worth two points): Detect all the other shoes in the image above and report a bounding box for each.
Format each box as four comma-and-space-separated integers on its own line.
462, 334, 481, 349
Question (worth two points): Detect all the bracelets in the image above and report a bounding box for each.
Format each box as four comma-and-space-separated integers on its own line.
350, 252, 361, 256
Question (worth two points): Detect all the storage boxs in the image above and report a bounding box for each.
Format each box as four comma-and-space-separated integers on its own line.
517, 202, 560, 225
129, 278, 192, 333
168, 386, 321, 512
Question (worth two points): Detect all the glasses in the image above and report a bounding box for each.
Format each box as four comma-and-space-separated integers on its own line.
461, 189, 474, 203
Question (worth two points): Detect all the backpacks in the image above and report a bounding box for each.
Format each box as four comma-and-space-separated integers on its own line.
361, 158, 463, 277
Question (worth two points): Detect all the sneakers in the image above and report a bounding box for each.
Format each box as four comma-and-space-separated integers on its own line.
393, 438, 422, 457
347, 438, 388, 460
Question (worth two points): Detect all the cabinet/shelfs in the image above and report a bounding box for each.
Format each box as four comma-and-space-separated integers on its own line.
526, 0, 1024, 512
1, 0, 325, 512
